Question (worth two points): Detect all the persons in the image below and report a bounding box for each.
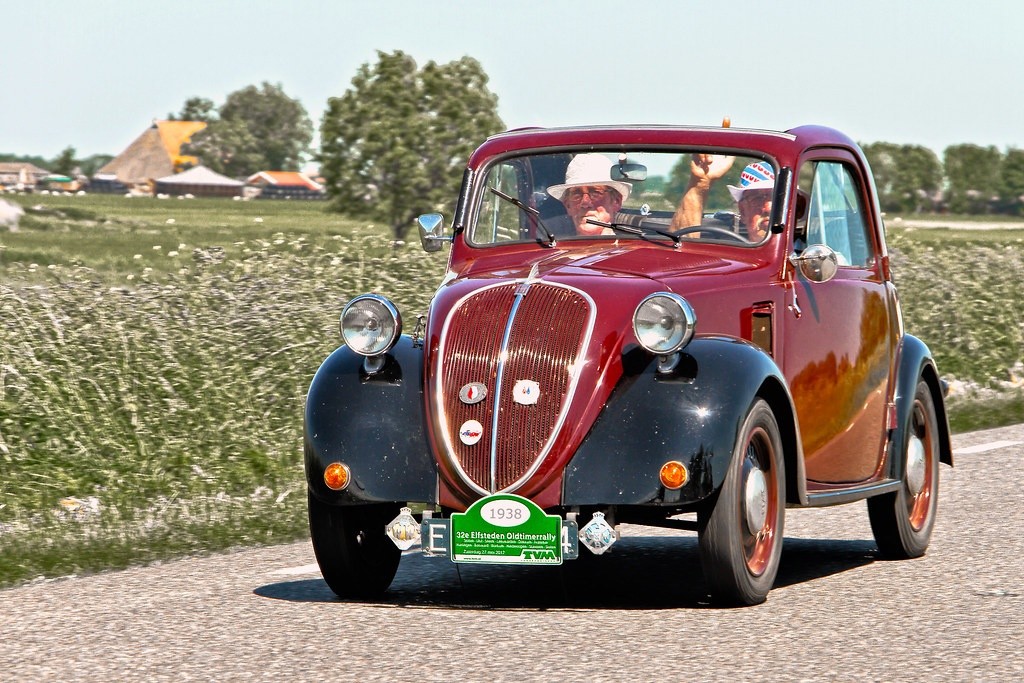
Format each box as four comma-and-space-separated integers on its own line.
546, 117, 810, 257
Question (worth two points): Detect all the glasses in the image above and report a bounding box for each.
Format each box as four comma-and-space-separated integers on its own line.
567, 189, 615, 202
739, 193, 773, 205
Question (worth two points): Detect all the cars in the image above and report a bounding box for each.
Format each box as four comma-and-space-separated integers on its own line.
303, 123, 954, 608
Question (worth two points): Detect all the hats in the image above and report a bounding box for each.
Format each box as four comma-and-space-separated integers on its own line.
546, 152, 632, 202
727, 162, 811, 222
